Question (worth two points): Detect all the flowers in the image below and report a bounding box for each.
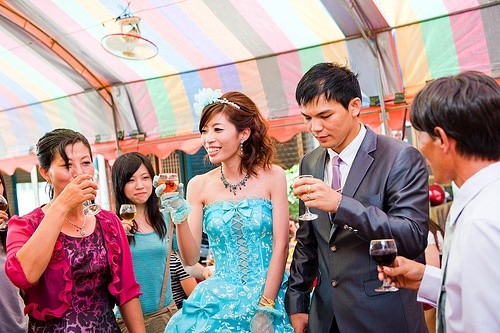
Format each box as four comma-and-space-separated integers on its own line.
193, 88, 223, 117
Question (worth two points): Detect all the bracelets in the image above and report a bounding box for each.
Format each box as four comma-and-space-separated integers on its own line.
258, 294, 275, 306
259, 302, 269, 307
179, 216, 188, 225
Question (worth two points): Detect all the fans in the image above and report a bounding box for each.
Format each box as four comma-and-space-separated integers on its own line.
100, 13, 159, 62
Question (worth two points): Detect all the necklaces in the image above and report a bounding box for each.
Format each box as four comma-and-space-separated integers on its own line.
50, 206, 86, 236
219, 162, 251, 196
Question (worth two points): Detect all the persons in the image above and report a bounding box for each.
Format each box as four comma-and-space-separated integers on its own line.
0, 61, 500, 333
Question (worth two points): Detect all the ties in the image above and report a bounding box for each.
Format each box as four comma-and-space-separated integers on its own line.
328, 155, 342, 221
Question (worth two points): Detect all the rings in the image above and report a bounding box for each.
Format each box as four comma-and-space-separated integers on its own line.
307, 184, 311, 193
307, 193, 310, 200
